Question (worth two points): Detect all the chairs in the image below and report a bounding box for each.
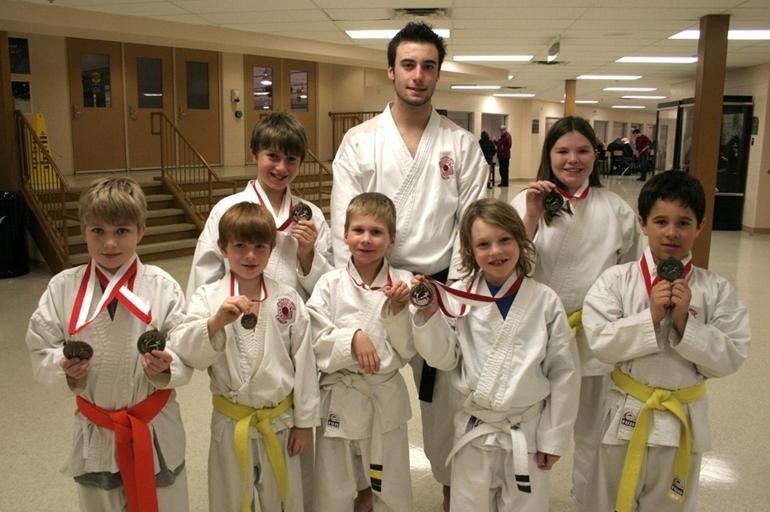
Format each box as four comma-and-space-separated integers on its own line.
611, 146, 635, 177
647, 139, 657, 176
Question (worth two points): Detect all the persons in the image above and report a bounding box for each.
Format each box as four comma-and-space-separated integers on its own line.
478, 131, 497, 189
607, 129, 651, 181
26, 176, 187, 512
329, 21, 491, 291
581, 170, 753, 512
168, 201, 322, 512
510, 116, 649, 512
492, 125, 512, 187
305, 191, 417, 512
186, 111, 335, 312
409, 198, 581, 512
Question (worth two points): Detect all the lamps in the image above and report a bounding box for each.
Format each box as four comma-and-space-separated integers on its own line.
261, 67, 272, 87
298, 87, 308, 101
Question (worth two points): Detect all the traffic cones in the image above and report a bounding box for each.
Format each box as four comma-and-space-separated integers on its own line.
26, 111, 60, 188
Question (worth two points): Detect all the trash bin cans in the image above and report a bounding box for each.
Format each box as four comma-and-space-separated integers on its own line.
0, 191, 30, 279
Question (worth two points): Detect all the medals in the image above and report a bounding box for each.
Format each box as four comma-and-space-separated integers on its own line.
656, 254, 684, 283
240, 312, 258, 330
543, 188, 564, 213
137, 329, 166, 359
62, 341, 95, 362
409, 282, 435, 309
291, 202, 312, 224
